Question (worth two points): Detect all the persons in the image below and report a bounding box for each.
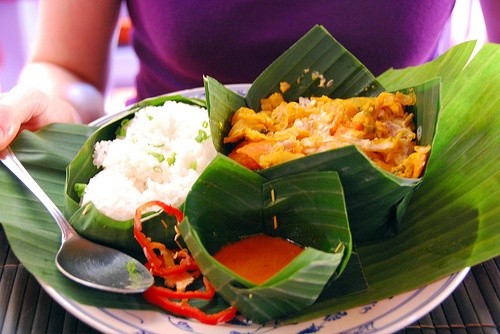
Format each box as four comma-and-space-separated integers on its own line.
1, 0, 500, 149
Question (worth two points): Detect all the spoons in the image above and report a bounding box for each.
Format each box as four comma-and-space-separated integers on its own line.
1, 144, 153, 295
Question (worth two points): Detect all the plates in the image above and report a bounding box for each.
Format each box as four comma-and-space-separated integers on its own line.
25, 83, 480, 333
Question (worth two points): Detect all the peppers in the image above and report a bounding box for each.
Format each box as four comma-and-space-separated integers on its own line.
133, 200, 238, 326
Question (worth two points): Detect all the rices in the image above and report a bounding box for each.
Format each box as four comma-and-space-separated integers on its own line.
79, 98, 216, 220
